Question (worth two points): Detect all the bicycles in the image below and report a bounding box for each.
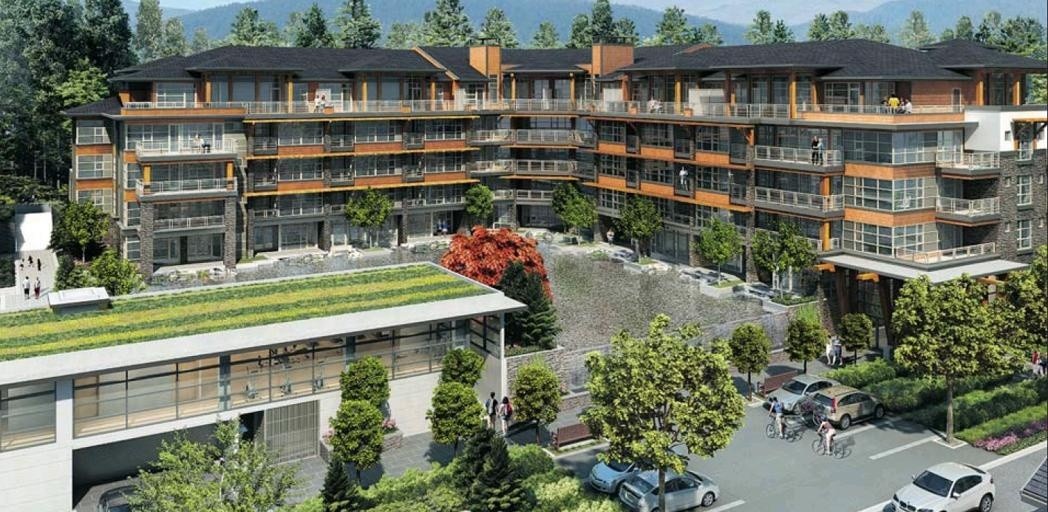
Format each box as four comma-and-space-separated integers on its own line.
812, 429, 845, 459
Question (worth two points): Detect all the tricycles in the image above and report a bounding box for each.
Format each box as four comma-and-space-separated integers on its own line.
765, 414, 807, 443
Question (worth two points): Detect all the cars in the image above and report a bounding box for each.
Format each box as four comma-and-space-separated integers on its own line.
807, 384, 885, 429
588, 446, 678, 494
619, 466, 719, 512
881, 462, 996, 512
763, 373, 841, 412
